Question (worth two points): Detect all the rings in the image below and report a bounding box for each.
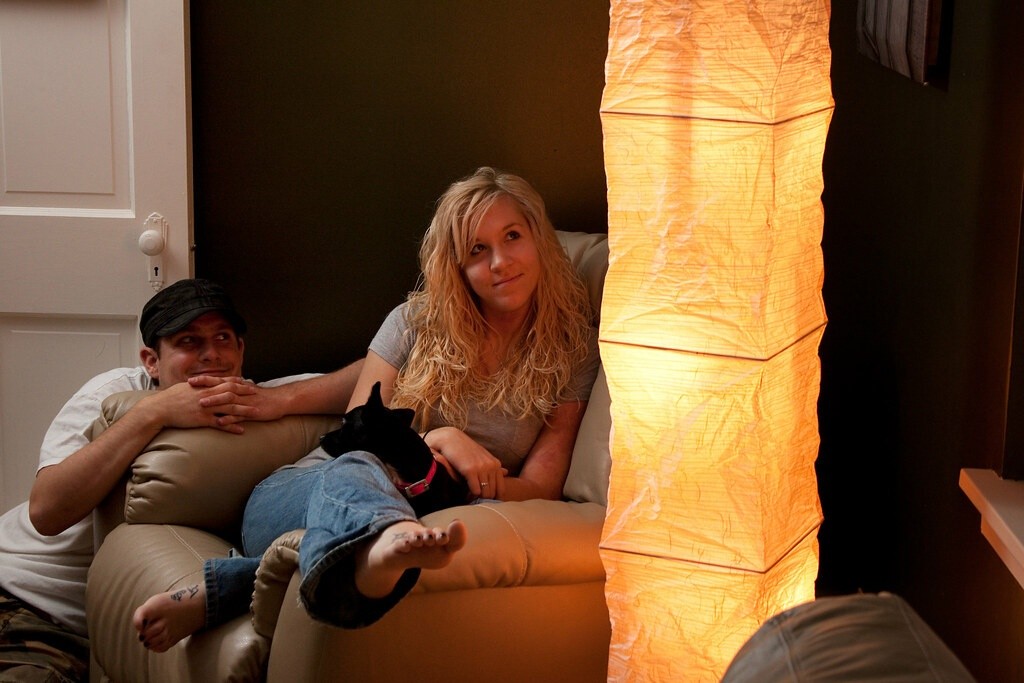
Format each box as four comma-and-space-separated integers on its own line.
481, 483, 489, 488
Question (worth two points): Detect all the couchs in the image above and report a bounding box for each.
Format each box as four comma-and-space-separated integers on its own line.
84, 229, 612, 683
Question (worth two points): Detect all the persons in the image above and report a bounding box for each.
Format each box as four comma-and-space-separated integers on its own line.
133, 166, 599, 655
0, 279, 365, 683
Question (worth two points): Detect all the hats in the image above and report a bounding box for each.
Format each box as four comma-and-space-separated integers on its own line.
140, 278, 240, 348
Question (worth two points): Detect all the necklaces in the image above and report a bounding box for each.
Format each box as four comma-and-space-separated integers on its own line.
483, 319, 534, 366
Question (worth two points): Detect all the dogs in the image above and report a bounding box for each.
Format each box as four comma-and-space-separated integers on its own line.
318, 380, 472, 518
